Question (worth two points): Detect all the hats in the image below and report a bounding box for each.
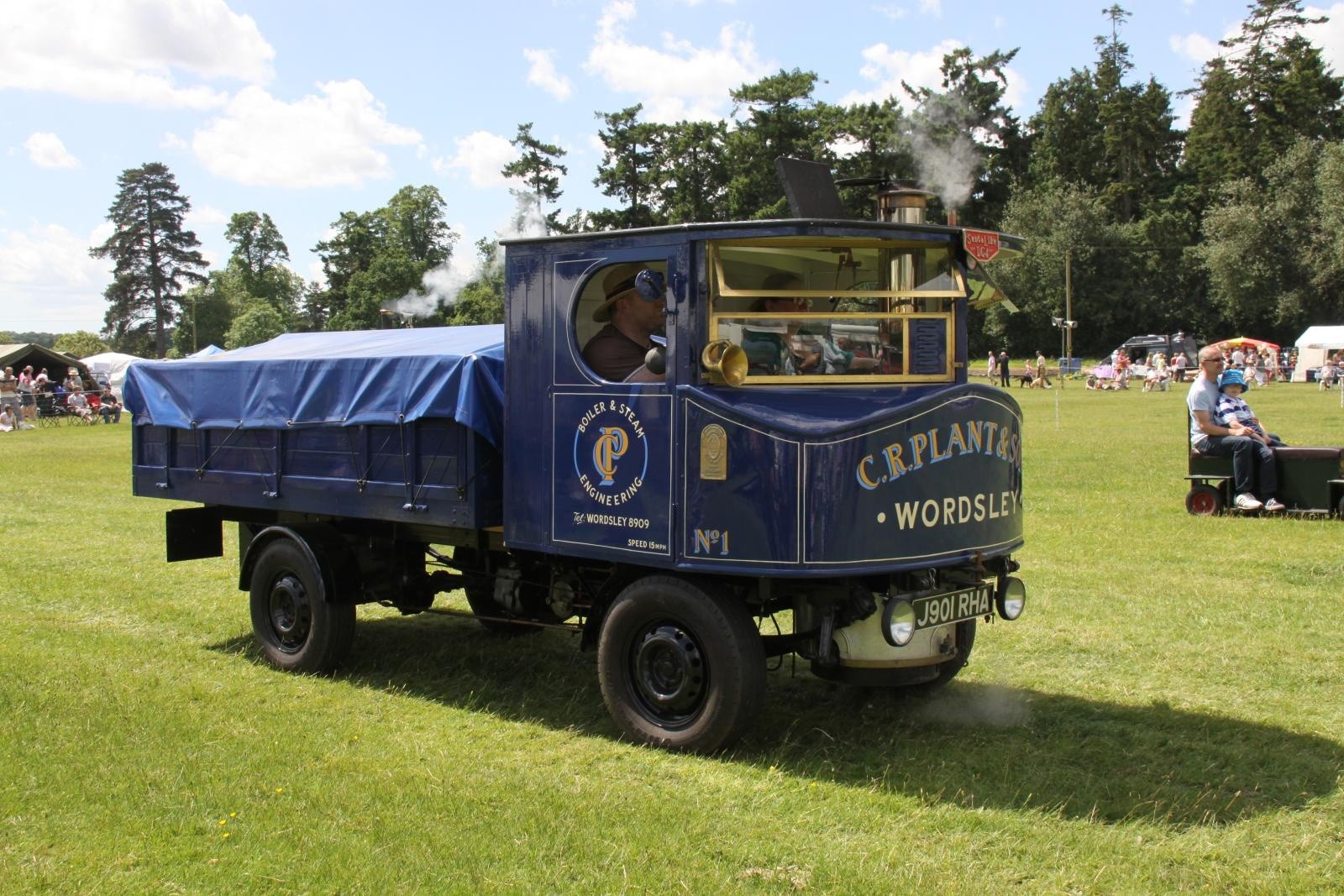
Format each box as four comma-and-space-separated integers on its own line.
1112, 350, 1119, 354
749, 274, 800, 311
68, 371, 78, 376
1218, 371, 1247, 393
592, 264, 668, 323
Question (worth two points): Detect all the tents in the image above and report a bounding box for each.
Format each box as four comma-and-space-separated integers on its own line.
1198, 335, 1283, 375
1290, 325, 1344, 382
78, 352, 149, 412
0, 343, 89, 404
1099, 331, 1198, 370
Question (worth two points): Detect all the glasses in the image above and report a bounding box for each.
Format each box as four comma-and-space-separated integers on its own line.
1203, 358, 1223, 362
772, 297, 814, 307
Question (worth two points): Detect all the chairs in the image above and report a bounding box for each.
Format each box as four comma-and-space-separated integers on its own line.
1187, 406, 1234, 476
92, 397, 122, 425
37, 394, 60, 428
64, 404, 90, 427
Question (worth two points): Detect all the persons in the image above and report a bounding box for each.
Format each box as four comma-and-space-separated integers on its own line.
1321, 352, 1344, 389
987, 351, 998, 386
0, 365, 121, 432
581, 261, 668, 382
1142, 350, 1170, 392
1214, 370, 1284, 511
1222, 346, 1274, 387
741, 271, 879, 377
1136, 357, 1145, 365
1185, 346, 1282, 511
1025, 359, 1033, 388
1036, 350, 1052, 389
1086, 349, 1133, 391
1171, 352, 1187, 383
998, 352, 1011, 387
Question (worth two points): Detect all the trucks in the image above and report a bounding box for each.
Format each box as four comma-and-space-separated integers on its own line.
132, 156, 1027, 755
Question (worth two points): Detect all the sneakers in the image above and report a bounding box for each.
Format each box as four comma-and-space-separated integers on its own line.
1263, 498, 1284, 510
1237, 493, 1263, 509
